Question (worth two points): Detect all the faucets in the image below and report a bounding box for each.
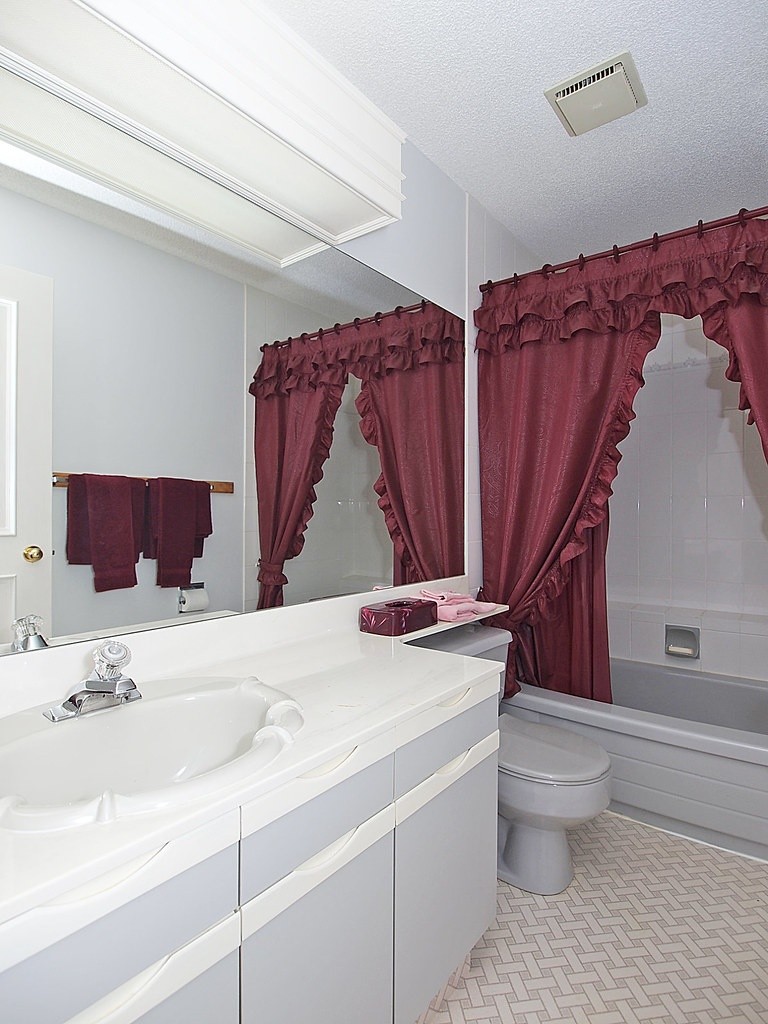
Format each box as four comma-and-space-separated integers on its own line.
39, 640, 145, 724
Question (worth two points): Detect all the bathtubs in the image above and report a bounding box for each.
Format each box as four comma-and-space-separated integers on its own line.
494, 651, 768, 867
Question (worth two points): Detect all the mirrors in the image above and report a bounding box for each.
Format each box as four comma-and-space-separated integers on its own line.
0, 61, 465, 661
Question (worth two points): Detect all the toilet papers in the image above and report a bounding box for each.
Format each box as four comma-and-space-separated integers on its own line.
180, 588, 211, 613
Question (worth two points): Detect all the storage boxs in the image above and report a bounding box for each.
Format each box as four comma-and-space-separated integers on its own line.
358, 597, 438, 636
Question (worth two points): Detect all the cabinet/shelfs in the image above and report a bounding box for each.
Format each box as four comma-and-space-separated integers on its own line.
395, 597, 513, 701
0, 68, 329, 268
393, 662, 509, 1024
0, 758, 239, 1024
239, 638, 393, 1023
1, 0, 408, 244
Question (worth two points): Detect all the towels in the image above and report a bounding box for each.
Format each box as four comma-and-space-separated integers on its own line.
419, 588, 498, 622
142, 477, 213, 588
65, 472, 148, 593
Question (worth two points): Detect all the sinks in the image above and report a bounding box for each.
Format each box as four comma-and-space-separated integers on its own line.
0, 676, 313, 838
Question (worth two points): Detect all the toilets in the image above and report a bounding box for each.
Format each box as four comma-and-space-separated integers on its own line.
405, 627, 612, 899
